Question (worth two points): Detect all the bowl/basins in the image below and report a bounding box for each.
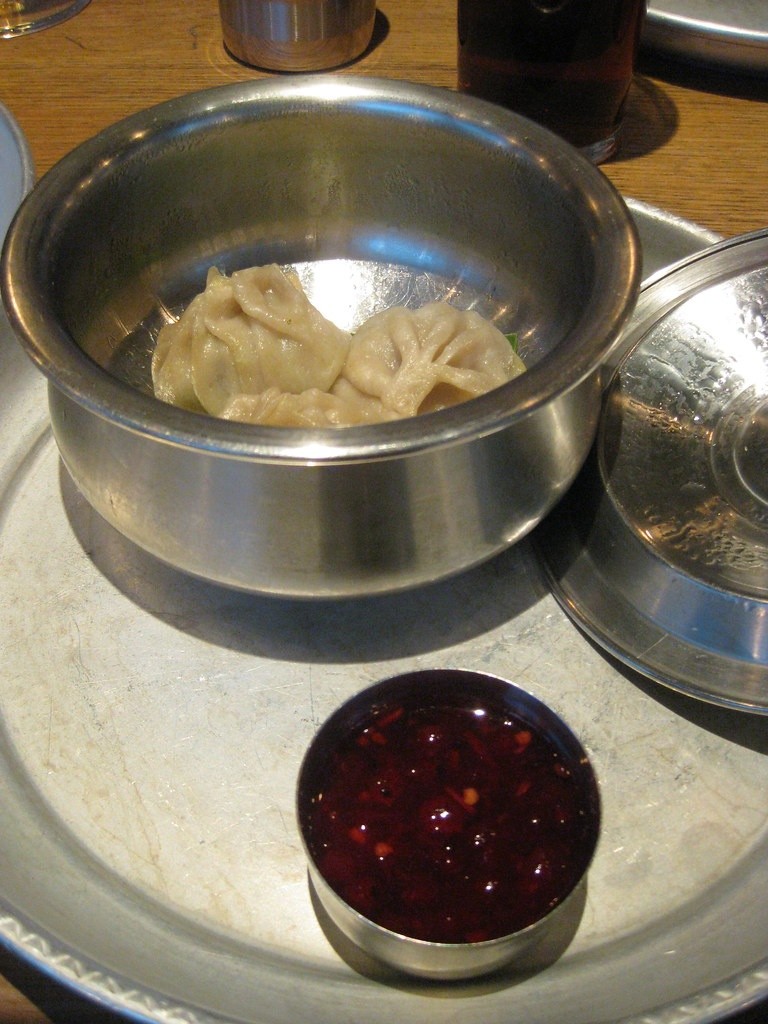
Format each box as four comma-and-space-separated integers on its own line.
2, 68, 646, 610
295, 667, 602, 987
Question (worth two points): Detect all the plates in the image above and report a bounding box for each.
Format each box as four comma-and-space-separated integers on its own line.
0, 191, 766, 1023
633, 1, 767, 70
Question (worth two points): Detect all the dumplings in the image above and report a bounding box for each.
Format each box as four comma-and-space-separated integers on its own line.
149, 256, 531, 429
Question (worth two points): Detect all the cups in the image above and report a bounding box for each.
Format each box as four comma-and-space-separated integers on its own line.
454, 0, 648, 164
217, 0, 378, 74
